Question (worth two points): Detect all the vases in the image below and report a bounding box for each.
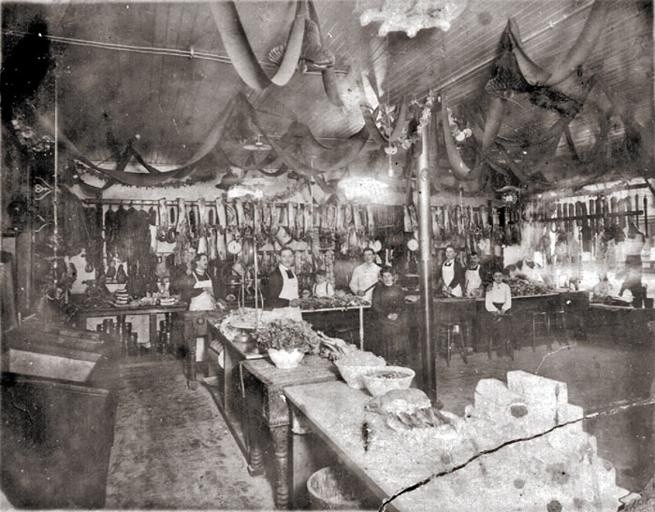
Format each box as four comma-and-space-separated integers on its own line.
360, 366, 415, 398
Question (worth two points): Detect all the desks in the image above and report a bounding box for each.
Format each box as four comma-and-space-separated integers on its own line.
71, 289, 588, 391
284, 382, 630, 510
237, 356, 339, 476
205, 317, 270, 414
588, 301, 654, 347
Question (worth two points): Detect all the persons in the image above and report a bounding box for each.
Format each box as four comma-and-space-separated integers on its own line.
123, 235, 160, 351
486, 270, 511, 357
460, 251, 488, 352
350, 247, 383, 351
169, 250, 194, 354
436, 245, 463, 344
181, 253, 226, 391
373, 267, 406, 366
266, 247, 303, 321
295, 263, 336, 338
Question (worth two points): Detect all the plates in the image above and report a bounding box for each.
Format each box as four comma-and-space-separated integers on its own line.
360, 365, 416, 397
384, 407, 460, 442
332, 355, 387, 391
267, 348, 306, 370
